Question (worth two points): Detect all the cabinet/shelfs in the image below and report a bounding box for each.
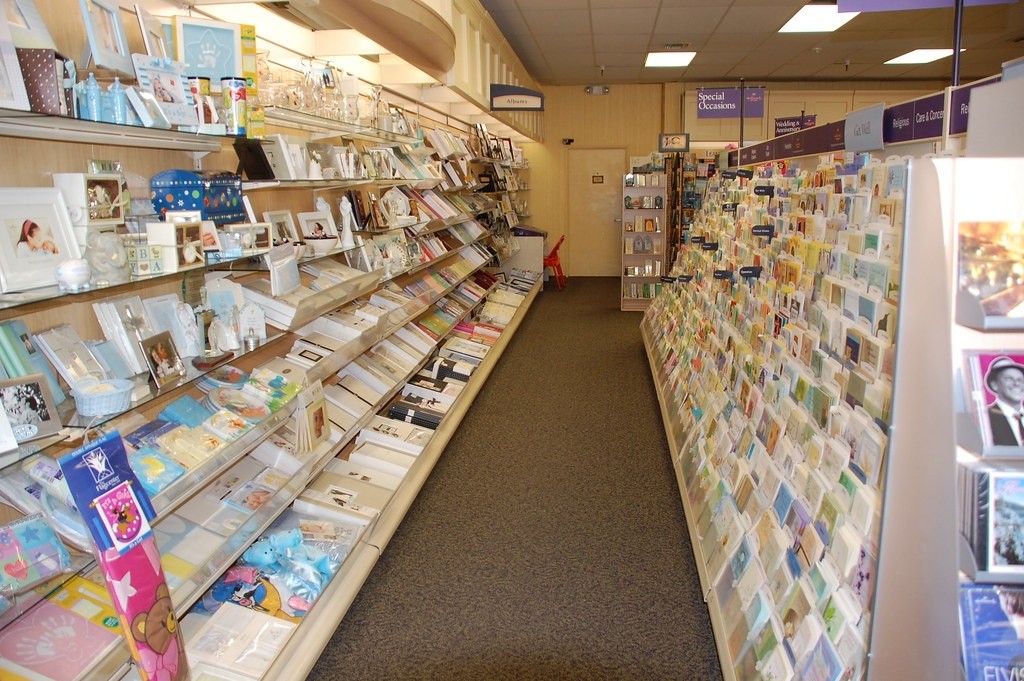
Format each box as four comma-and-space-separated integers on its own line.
0, 0, 541, 681
620, 158, 1024, 681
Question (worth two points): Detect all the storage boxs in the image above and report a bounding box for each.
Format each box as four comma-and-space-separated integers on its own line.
74, 225, 116, 254
53, 172, 124, 226
171, 14, 258, 96
224, 222, 273, 253
16, 48, 74, 118
146, 221, 204, 272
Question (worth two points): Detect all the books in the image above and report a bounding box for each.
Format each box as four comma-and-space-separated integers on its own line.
645, 153, 1024, 681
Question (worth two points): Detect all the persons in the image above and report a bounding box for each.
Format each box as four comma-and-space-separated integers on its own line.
311, 223, 323, 235
984, 356, 1024, 446
17, 221, 60, 256
149, 342, 176, 378
313, 408, 324, 437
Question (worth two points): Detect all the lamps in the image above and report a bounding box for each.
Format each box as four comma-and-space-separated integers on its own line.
585, 86, 610, 95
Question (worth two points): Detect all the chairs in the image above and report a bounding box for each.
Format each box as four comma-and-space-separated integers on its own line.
543, 235, 565, 290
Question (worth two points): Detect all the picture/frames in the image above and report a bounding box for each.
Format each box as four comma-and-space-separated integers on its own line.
138, 330, 187, 390
0, 373, 63, 444
261, 134, 294, 180
288, 144, 307, 179
262, 209, 299, 243
135, 4, 171, 59
0, 187, 82, 294
80, 0, 135, 79
232, 143, 275, 182
132, 53, 200, 126
0, 0, 59, 52
0, 0, 31, 111
296, 212, 342, 248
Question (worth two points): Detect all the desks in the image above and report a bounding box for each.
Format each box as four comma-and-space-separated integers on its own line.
500, 234, 549, 291
